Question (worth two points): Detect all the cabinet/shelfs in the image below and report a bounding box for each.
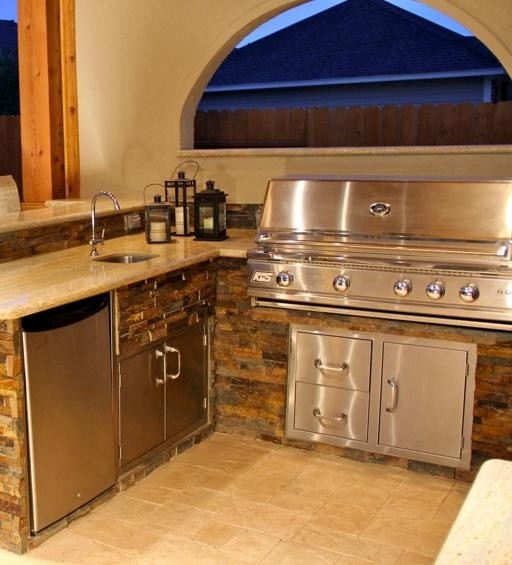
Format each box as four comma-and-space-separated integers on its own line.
118, 324, 206, 469
286, 321, 372, 450
372, 337, 478, 475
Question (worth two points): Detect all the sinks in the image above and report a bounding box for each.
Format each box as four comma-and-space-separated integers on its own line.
91, 252, 161, 265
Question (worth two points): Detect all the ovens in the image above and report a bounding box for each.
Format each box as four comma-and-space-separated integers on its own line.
20, 291, 119, 535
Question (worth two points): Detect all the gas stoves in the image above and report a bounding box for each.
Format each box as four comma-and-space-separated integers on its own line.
256, 227, 511, 325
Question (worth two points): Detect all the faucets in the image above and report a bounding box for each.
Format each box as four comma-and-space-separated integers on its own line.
88, 189, 121, 256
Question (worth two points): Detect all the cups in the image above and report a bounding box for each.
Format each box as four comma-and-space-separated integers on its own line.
174, 206, 190, 235
